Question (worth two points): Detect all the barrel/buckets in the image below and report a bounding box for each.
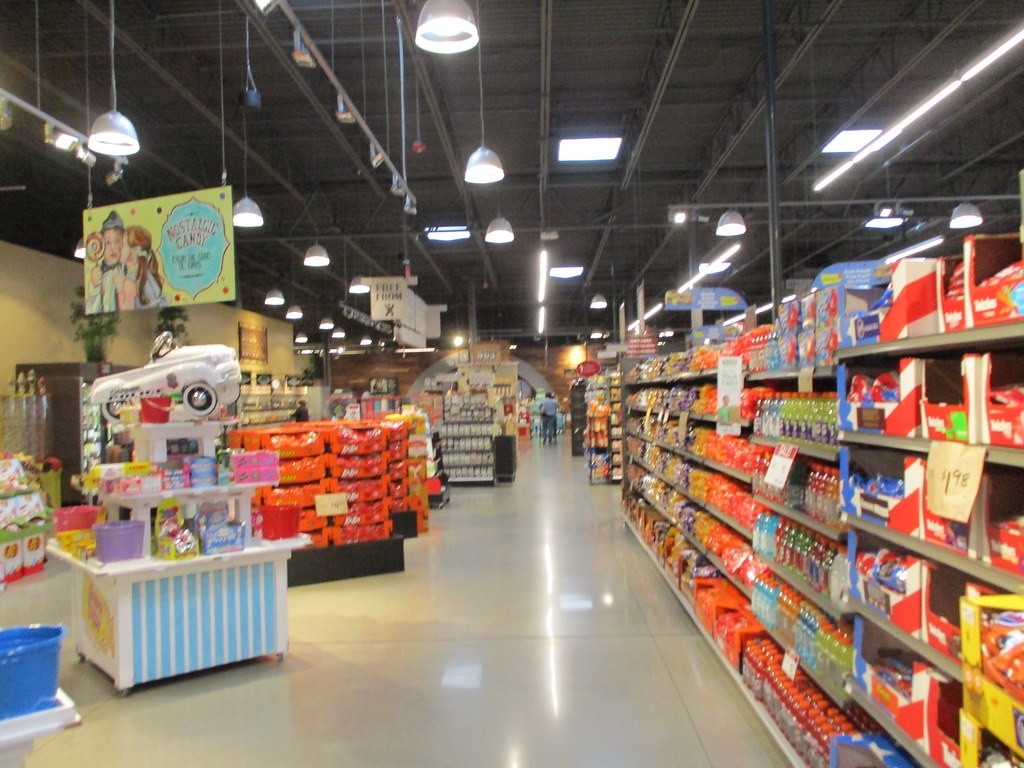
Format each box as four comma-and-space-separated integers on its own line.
0, 624, 65, 721
93, 519, 145, 564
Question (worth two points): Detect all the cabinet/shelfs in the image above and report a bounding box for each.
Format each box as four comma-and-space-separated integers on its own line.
240, 407, 297, 427
46, 416, 315, 697
829, 321, 1024, 768
606, 376, 623, 486
440, 406, 497, 488
614, 349, 848, 768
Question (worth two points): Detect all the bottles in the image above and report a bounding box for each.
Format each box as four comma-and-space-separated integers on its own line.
736, 385, 885, 767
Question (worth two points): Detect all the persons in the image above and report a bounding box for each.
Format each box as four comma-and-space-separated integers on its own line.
538, 392, 559, 446
290, 400, 309, 422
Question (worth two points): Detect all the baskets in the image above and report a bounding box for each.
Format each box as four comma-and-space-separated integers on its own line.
94, 519, 145, 562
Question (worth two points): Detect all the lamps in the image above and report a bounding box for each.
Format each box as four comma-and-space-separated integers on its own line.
285, 304, 304, 320
331, 326, 346, 338
87, 0, 140, 156
590, 293, 607, 309
289, 0, 424, 217
485, 196, 514, 243
49, 131, 80, 151
264, 289, 285, 306
294, 331, 308, 343
319, 316, 334, 330
464, 0, 504, 184
107, 170, 123, 185
348, 274, 371, 295
232, 109, 264, 227
302, 234, 330, 267
242, 15, 262, 108
75, 148, 97, 168
360, 334, 373, 346
415, 0, 479, 54
715, 210, 746, 236
949, 202, 983, 229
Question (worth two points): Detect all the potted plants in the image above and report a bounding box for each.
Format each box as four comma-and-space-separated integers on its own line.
66, 285, 121, 363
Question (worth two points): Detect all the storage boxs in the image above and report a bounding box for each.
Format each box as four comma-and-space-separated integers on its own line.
440, 409, 493, 478
92, 413, 437, 561
0, 458, 44, 585
774, 236, 1024, 768
634, 504, 768, 675
